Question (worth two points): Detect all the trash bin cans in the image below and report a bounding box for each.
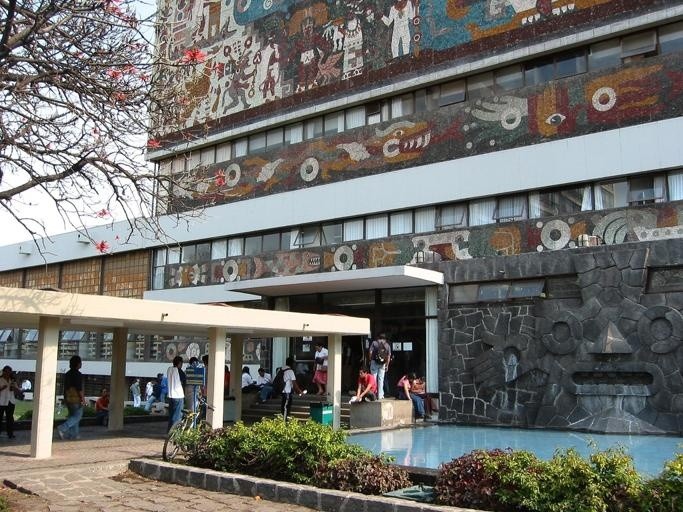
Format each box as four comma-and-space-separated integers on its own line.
310, 401, 333, 428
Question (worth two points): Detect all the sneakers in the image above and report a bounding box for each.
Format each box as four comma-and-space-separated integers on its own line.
56, 429, 65, 439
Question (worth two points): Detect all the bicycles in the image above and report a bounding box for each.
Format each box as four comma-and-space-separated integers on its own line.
161, 394, 215, 462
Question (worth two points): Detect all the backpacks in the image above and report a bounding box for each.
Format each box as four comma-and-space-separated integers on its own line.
375, 340, 388, 364
272, 367, 292, 393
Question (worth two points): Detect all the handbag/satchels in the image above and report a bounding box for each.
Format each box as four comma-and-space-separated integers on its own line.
66, 388, 83, 403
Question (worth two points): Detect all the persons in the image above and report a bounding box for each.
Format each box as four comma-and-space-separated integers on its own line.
274, 357, 305, 424
396, 373, 426, 423
129, 372, 167, 411
349, 335, 391, 402
242, 366, 274, 405
313, 343, 328, 396
55, 355, 85, 441
166, 354, 209, 430
225, 364, 230, 395
95, 387, 109, 427
0, 365, 18, 438
21, 379, 32, 394
